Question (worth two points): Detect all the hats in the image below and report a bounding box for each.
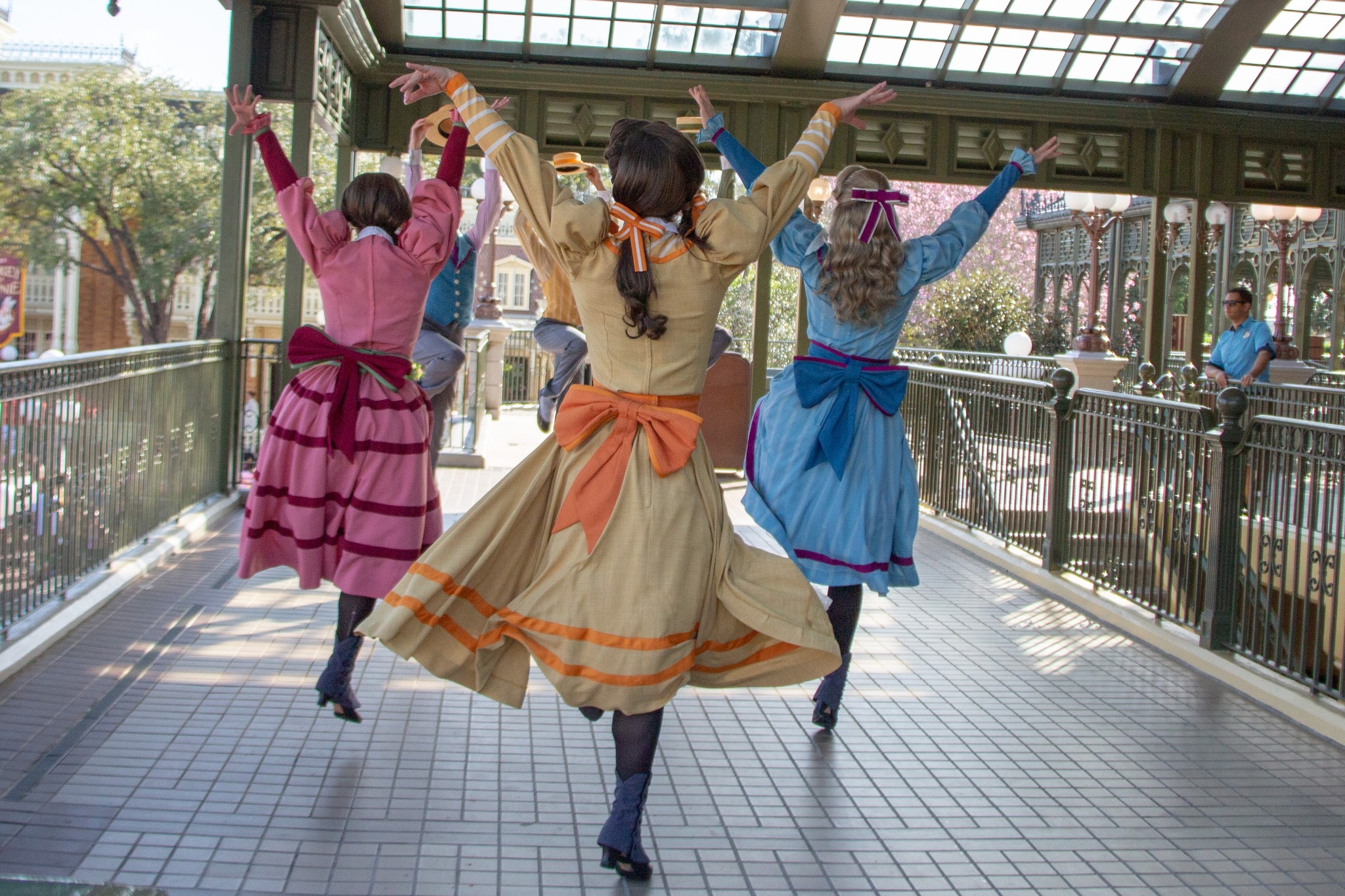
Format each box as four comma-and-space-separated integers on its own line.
423, 102, 480, 149
550, 151, 593, 176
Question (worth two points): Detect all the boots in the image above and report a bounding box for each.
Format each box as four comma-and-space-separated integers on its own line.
597, 767, 652, 880
314, 632, 365, 725
811, 652, 852, 727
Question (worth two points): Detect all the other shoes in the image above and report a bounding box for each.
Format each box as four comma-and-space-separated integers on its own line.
537, 389, 556, 434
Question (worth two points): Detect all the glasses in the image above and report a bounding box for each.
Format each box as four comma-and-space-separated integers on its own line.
1222, 300, 1245, 305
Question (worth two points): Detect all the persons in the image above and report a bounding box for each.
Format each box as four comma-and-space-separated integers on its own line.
685, 82, 1062, 730
1205, 287, 1276, 511
675, 185, 735, 377
222, 78, 514, 725
383, 56, 902, 884
514, 164, 618, 444
397, 94, 513, 569
